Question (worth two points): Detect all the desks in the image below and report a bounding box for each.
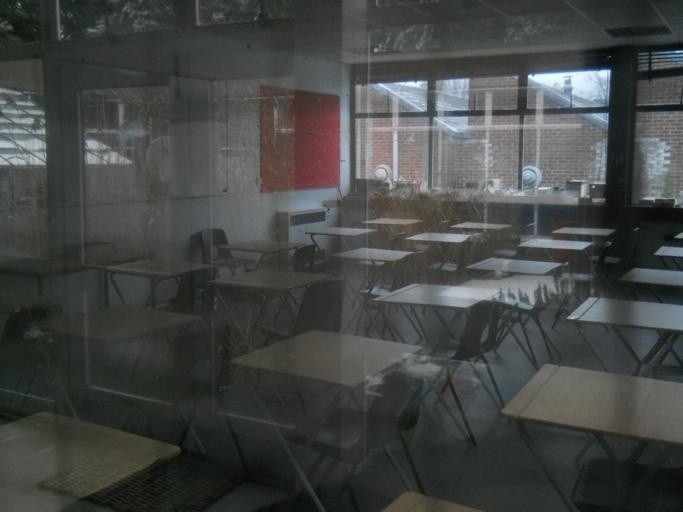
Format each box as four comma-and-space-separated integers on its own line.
0, 191, 683, 512
